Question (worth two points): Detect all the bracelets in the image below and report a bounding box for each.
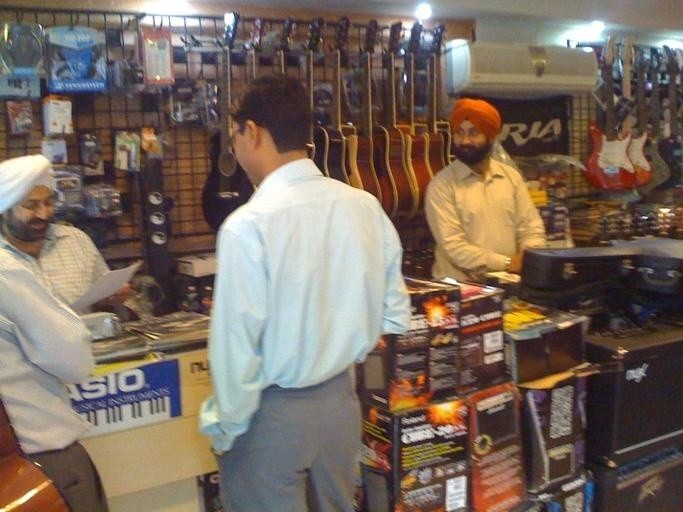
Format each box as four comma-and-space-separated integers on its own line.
504, 257, 512, 271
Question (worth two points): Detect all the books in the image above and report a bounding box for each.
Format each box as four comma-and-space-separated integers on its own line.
91, 310, 210, 364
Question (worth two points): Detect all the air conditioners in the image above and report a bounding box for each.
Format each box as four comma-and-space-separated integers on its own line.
445, 40, 600, 98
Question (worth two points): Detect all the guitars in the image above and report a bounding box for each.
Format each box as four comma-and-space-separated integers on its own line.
584, 34, 682, 194
246, 17, 291, 82
200, 12, 257, 231
306, 19, 451, 221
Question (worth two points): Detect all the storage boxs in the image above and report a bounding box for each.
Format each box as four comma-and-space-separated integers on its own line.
347, 275, 683, 512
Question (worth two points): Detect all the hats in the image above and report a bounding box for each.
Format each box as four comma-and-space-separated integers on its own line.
449, 97, 501, 136
0, 154, 57, 213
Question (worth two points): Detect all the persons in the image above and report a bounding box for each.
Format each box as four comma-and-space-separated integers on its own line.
1, 248, 108, 512
200, 74, 411, 512
423, 98, 546, 284
0, 153, 133, 317
